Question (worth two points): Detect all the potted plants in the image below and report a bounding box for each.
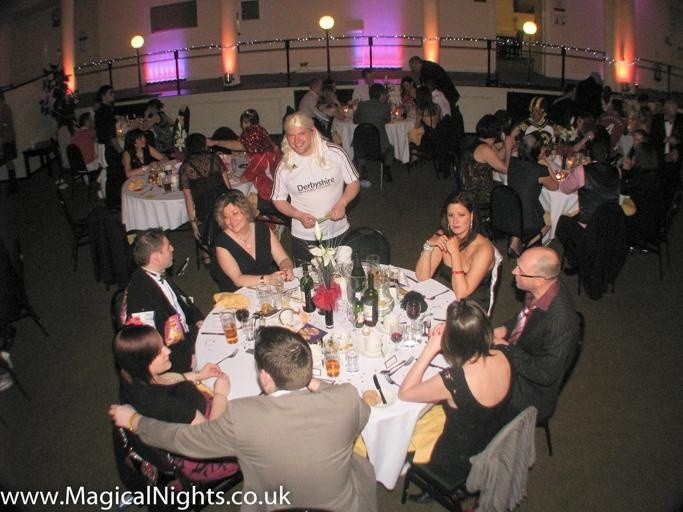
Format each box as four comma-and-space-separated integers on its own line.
38, 62, 74, 170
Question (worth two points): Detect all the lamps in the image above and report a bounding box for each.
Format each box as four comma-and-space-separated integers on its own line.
319, 15, 337, 31
523, 20, 538, 37
131, 35, 144, 50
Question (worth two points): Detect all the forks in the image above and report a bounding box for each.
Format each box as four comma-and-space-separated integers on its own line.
214, 348, 239, 369
390, 355, 414, 376
385, 374, 401, 389
426, 289, 449, 300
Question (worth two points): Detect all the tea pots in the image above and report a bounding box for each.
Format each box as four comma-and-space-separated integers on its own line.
377, 278, 402, 317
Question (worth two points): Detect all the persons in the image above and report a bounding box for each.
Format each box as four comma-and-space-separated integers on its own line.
397, 298, 517, 503
621, 142, 667, 241
400, 76, 416, 100
143, 98, 174, 154
270, 111, 360, 268
352, 68, 375, 110
492, 247, 581, 422
648, 99, 683, 215
127, 228, 204, 371
494, 71, 652, 158
69, 111, 100, 173
178, 134, 231, 240
349, 83, 394, 180
507, 134, 559, 263
112, 324, 240, 482
297, 76, 323, 119
312, 79, 345, 142
409, 87, 444, 147
206, 108, 279, 157
408, 56, 460, 108
118, 128, 167, 191
239, 124, 275, 215
421, 78, 452, 119
459, 114, 516, 211
93, 85, 122, 213
415, 191, 495, 313
108, 326, 377, 512
555, 141, 622, 276
212, 189, 295, 288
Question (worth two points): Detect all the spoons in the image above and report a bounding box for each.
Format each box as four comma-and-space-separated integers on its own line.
380, 360, 405, 374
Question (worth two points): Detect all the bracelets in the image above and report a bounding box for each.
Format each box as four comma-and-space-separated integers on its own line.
451, 271, 464, 275
423, 243, 433, 251
259, 273, 265, 285
193, 371, 200, 384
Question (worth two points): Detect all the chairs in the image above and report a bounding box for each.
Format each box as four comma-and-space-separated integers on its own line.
401, 407, 538, 512
487, 184, 543, 258
0, 323, 34, 427
352, 123, 402, 193
567, 205, 621, 297
6, 239, 51, 337
109, 410, 241, 511
452, 159, 506, 247
54, 176, 105, 269
623, 191, 682, 282
352, 225, 393, 267
66, 143, 104, 201
527, 311, 585, 456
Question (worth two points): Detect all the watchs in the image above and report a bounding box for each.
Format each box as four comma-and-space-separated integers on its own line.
128, 412, 139, 434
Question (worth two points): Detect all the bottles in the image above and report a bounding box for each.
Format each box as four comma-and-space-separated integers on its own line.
363, 273, 379, 328
353, 288, 365, 329
299, 262, 316, 313
350, 248, 367, 303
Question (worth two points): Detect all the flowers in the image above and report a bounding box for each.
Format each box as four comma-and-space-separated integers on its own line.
174, 122, 186, 152
309, 221, 353, 277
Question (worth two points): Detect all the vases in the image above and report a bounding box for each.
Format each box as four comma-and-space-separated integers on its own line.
174, 152, 186, 164
324, 308, 335, 329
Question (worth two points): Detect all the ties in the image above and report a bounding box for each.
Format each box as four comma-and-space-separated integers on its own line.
507, 307, 530, 346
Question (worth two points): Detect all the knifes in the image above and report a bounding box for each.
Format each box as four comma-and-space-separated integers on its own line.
372, 374, 387, 406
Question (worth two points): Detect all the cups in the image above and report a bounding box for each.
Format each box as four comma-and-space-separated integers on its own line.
275, 277, 285, 293
390, 323, 403, 344
412, 320, 423, 343
258, 292, 273, 312
220, 313, 238, 344
404, 325, 417, 348
367, 254, 380, 276
576, 154, 582, 165
242, 318, 254, 342
321, 340, 341, 378
257, 285, 271, 303
162, 177, 172, 192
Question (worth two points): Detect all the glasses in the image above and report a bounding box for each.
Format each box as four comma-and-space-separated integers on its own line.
516, 258, 543, 279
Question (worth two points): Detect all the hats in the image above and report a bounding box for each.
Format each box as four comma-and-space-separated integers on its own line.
529, 96, 547, 110
